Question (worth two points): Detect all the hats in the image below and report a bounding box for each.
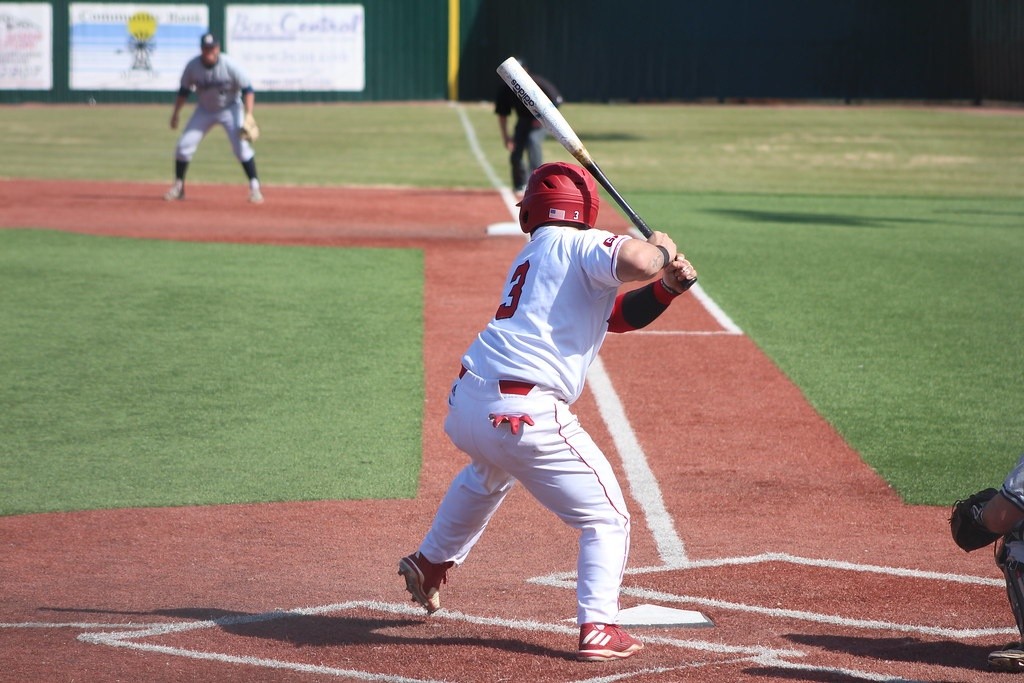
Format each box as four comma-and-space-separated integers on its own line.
202, 33, 220, 49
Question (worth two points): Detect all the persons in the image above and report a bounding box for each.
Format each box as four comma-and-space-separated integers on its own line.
493, 60, 562, 197
950, 455, 1024, 672
164, 33, 264, 202
398, 162, 697, 662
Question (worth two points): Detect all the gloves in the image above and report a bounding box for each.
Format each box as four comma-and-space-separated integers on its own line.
488, 413, 535, 435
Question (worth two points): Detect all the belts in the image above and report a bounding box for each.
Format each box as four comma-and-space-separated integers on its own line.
458, 361, 536, 396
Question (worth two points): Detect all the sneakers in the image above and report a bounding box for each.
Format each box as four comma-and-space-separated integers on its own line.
397, 551, 454, 615
577, 624, 646, 662
988, 641, 1024, 670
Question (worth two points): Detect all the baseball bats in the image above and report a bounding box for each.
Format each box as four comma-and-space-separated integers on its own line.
496, 56, 699, 290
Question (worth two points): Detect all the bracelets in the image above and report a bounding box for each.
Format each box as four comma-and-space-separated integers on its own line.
656, 245, 670, 269
661, 278, 681, 295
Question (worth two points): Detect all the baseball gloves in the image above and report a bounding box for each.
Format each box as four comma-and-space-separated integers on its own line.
948, 486, 999, 553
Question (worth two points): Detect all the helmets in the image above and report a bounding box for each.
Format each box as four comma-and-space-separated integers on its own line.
515, 162, 599, 234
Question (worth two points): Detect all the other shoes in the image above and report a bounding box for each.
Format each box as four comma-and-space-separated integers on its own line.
250, 187, 264, 205
163, 186, 185, 201
